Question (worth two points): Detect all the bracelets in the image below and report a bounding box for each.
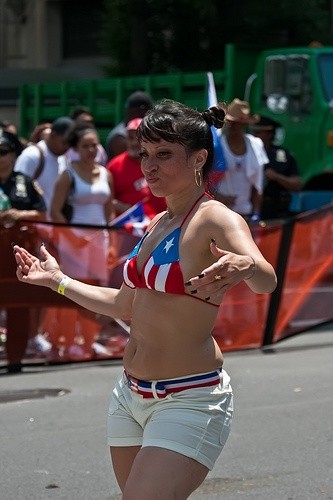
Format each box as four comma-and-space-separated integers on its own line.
250, 212, 261, 223
245, 254, 256, 280
58, 276, 71, 295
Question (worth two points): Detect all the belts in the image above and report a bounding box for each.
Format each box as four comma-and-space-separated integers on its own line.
123, 367, 225, 400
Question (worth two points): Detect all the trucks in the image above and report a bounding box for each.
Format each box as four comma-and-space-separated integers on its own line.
17, 45, 333, 193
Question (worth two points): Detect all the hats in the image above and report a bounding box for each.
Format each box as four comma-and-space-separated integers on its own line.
217, 98, 261, 125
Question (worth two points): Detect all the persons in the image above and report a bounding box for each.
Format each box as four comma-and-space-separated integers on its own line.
0, 91, 299, 358
11, 97, 278, 500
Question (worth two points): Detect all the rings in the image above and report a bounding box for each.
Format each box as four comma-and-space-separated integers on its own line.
215, 274, 221, 280
